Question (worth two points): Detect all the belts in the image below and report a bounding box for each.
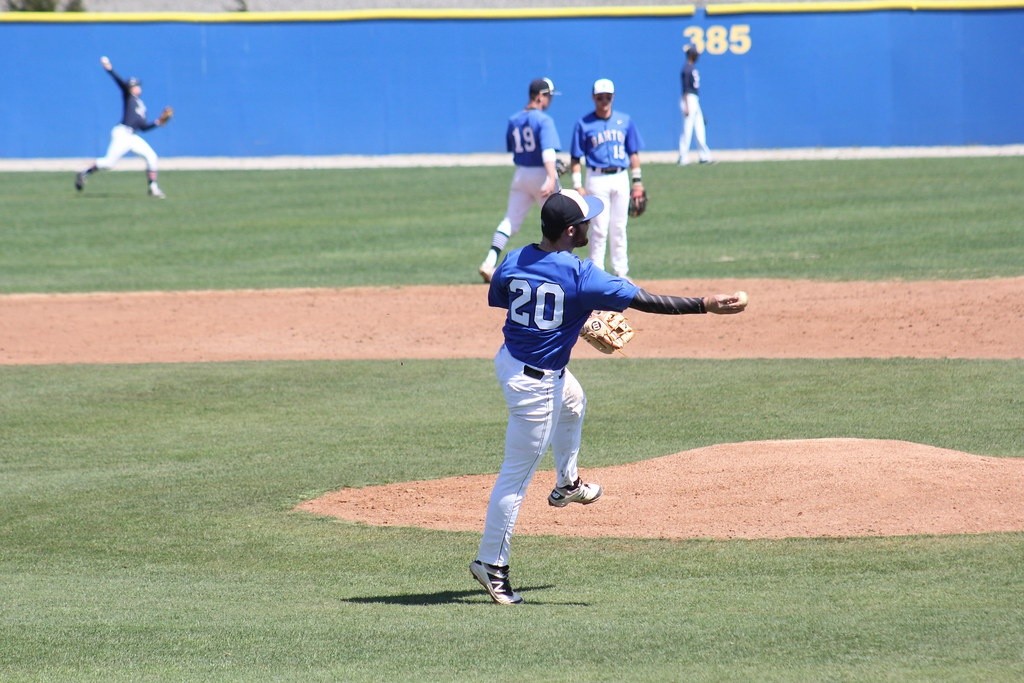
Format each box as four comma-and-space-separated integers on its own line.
590, 166, 625, 175
523, 365, 565, 380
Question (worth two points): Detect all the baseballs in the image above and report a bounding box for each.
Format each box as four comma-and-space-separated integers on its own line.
734, 290, 748, 305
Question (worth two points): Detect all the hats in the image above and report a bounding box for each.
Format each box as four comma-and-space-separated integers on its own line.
687, 47, 698, 55
541, 187, 604, 230
592, 79, 615, 95
126, 78, 140, 88
530, 77, 561, 96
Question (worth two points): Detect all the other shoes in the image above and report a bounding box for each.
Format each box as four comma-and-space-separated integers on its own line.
698, 155, 714, 162
478, 263, 496, 282
76, 172, 85, 190
679, 159, 688, 164
148, 186, 160, 193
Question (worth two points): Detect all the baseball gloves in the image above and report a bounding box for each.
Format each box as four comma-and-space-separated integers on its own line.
627, 186, 648, 218
159, 105, 172, 124
579, 306, 633, 356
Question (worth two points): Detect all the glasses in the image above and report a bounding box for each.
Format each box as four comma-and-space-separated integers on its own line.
595, 97, 613, 100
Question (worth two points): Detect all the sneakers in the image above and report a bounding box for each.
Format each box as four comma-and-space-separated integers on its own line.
548, 477, 603, 507
469, 562, 524, 605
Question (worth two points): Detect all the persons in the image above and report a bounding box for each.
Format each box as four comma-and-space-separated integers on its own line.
469, 188, 747, 604
479, 77, 561, 281
76, 57, 174, 198
569, 79, 648, 280
675, 49, 713, 163
